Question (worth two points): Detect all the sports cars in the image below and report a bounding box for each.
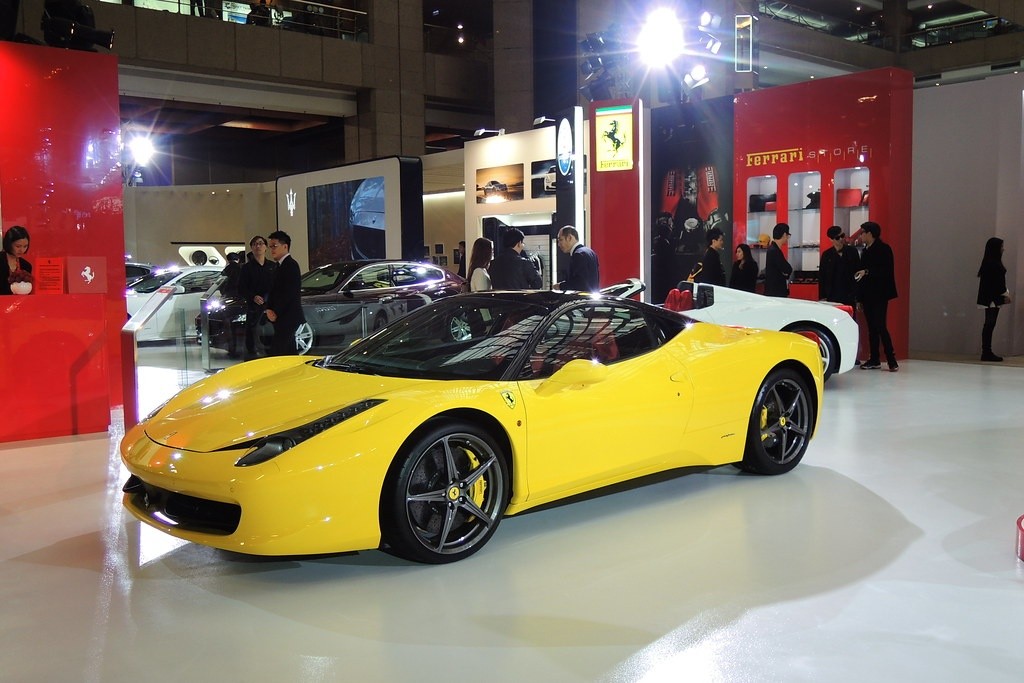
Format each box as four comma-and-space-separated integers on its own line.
596, 278, 859, 383
120, 291, 825, 564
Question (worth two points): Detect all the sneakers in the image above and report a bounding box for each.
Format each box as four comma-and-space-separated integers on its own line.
889, 360, 898, 371
860, 357, 881, 369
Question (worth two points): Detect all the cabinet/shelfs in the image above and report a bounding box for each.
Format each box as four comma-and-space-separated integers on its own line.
744, 163, 874, 283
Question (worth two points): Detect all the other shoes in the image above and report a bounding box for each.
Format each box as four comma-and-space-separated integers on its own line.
980, 349, 1003, 362
855, 360, 860, 365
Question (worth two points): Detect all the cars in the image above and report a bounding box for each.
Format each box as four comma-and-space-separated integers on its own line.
195, 259, 469, 355
125, 263, 224, 341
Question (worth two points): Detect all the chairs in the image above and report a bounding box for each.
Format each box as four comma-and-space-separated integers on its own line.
493, 312, 620, 374
201, 277, 212, 288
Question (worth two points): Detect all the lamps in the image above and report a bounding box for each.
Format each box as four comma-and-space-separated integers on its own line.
573, 7, 722, 101
122, 135, 154, 186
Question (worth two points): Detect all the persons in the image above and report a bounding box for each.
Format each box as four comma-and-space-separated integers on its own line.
693, 229, 726, 287
247, 0, 269, 26
765, 223, 793, 298
190, 0, 205, 17
731, 244, 759, 293
978, 237, 1011, 361
554, 227, 599, 292
0, 226, 32, 295
457, 241, 466, 279
221, 230, 308, 361
489, 228, 543, 289
854, 221, 898, 372
818, 226, 861, 365
467, 238, 491, 291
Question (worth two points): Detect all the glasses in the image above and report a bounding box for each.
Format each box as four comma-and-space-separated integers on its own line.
269, 243, 287, 249
786, 231, 791, 240
521, 241, 526, 247
251, 242, 266, 247
835, 232, 845, 240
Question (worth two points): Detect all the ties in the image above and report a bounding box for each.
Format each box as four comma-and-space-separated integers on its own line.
276, 262, 279, 269
